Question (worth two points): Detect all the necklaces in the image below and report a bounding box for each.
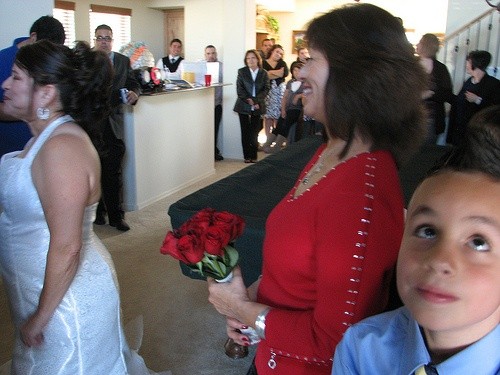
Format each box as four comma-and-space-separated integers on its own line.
303, 138, 337, 185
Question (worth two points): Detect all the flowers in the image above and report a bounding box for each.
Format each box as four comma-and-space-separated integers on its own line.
162, 208, 245, 280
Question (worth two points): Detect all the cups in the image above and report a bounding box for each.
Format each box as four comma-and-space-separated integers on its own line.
163, 67, 171, 81
204, 75, 212, 85
181, 71, 195, 82
120, 88, 129, 104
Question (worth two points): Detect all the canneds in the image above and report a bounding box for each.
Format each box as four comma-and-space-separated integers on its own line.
119, 88, 129, 104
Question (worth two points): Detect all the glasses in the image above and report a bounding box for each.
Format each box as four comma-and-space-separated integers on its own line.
93, 36, 113, 42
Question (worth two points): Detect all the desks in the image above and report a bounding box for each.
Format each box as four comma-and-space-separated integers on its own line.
110, 81, 233, 210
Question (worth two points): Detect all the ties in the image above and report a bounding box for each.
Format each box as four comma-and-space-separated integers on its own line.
171, 58, 175, 65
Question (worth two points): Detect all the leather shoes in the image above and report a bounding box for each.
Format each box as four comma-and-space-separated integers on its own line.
95, 212, 105, 225
108, 216, 130, 232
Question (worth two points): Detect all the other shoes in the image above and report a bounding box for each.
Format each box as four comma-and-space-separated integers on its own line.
245, 157, 255, 162
215, 148, 223, 161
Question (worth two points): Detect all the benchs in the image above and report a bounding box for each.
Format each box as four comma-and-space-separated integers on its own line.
168, 134, 323, 286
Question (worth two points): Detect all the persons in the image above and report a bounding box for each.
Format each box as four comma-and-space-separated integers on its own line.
421, 49, 500, 144
331, 143, 500, 375
259, 37, 289, 146
0, 14, 66, 156
293, 46, 328, 138
81, 23, 140, 230
200, 44, 224, 160
205, 2, 429, 374
0, 40, 129, 375
155, 37, 185, 80
257, 60, 304, 153
411, 30, 452, 143
233, 49, 270, 163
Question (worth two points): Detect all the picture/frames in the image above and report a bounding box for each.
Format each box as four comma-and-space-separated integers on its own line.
292, 31, 307, 54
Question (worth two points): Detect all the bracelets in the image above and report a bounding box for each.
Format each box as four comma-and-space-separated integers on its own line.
255, 306, 272, 339
